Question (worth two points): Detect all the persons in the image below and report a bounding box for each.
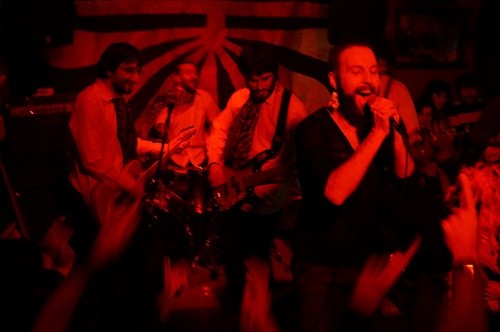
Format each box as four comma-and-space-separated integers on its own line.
68, 42, 168, 242
0, 216, 419, 332
415, 72, 500, 148
140, 62, 221, 168
205, 43, 309, 273
437, 145, 500, 332
293, 43, 421, 266
374, 47, 425, 158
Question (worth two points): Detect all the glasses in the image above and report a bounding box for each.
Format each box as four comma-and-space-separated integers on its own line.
119, 66, 141, 73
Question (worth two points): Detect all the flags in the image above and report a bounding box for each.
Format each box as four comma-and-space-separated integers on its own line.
68, 0, 336, 115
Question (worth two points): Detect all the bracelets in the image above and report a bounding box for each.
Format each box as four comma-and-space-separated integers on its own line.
453, 262, 474, 269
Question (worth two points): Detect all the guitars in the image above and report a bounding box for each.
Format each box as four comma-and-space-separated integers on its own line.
202, 164, 296, 223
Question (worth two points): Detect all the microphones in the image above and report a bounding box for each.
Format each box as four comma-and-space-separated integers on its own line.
389, 117, 409, 139
